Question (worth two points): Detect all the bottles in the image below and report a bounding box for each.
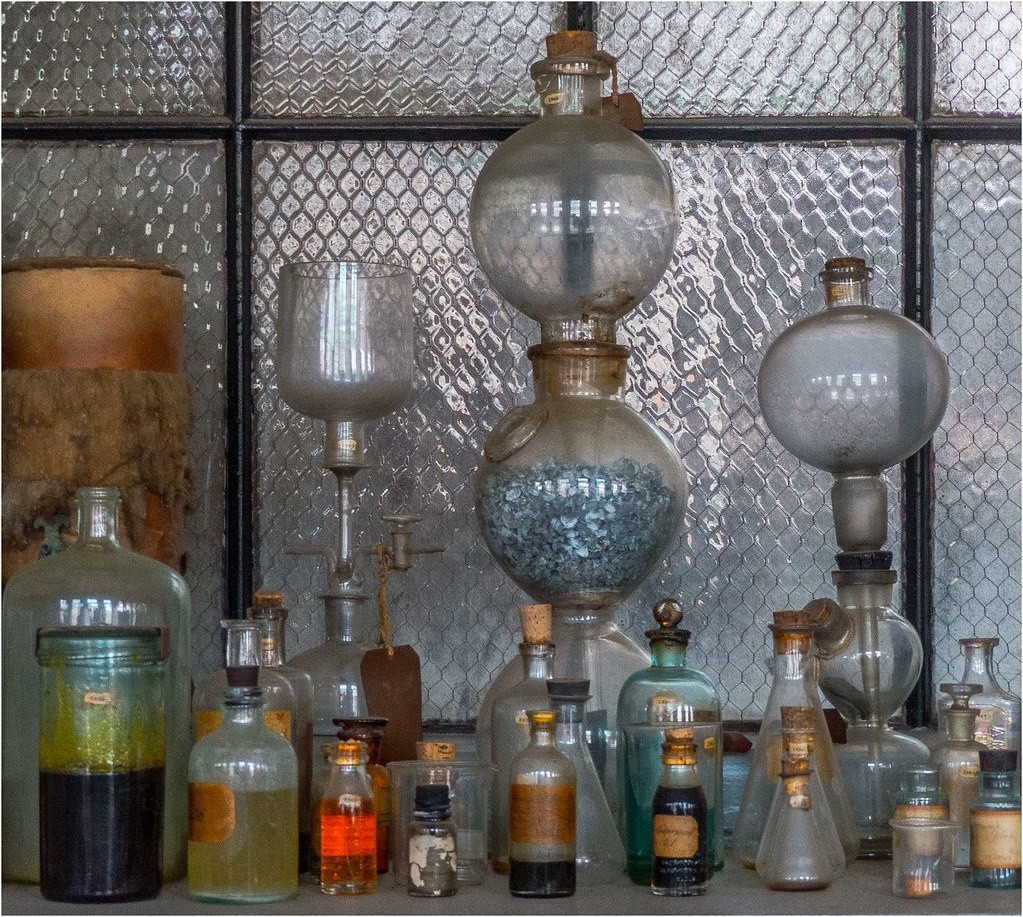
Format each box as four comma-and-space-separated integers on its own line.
937, 638, 1021, 799
928, 707, 988, 874
186, 618, 301, 905
544, 678, 625, 888
189, 619, 300, 761
617, 597, 726, 884
283, 568, 379, 874
1, 485, 189, 888
648, 740, 712, 896
406, 784, 460, 899
299, 737, 365, 765
893, 764, 953, 822
506, 710, 579, 899
733, 610, 861, 871
487, 603, 556, 875
753, 705, 847, 892
320, 741, 379, 897
968, 748, 1022, 891
234, 592, 315, 737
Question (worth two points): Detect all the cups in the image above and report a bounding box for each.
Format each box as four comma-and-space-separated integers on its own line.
890, 818, 962, 898
387, 761, 501, 886
